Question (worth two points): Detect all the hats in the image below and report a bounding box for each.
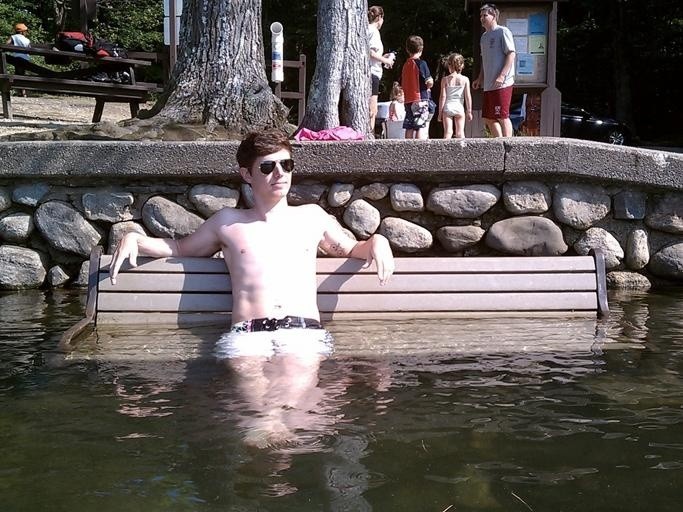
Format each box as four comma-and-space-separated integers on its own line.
16, 23, 28, 30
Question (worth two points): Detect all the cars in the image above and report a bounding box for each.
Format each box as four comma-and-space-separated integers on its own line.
508, 100, 632, 146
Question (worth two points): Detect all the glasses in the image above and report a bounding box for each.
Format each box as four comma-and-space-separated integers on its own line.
248, 159, 295, 175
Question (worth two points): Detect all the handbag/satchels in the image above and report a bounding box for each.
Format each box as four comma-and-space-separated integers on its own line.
57, 31, 94, 52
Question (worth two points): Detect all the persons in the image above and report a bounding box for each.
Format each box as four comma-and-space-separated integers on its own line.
5, 23, 31, 97
366, 5, 518, 140
108, 127, 396, 448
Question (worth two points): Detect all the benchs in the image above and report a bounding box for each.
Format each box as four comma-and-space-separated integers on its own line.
45, 249, 610, 363
0, 45, 158, 123
